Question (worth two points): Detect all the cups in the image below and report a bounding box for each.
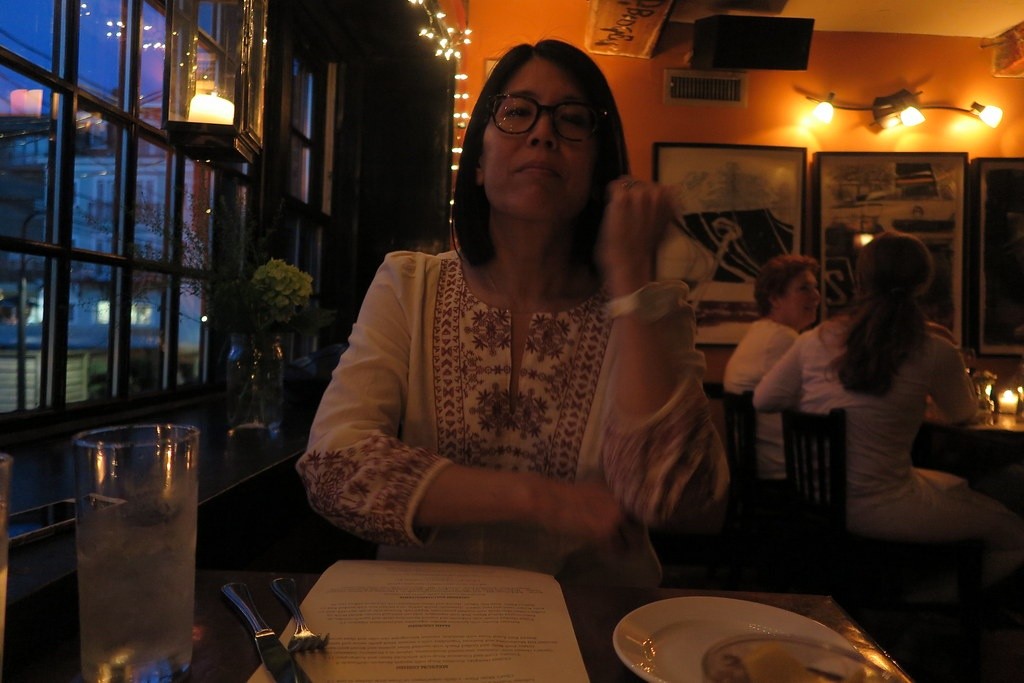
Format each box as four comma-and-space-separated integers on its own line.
73, 424, 200, 683
700, 632, 902, 683
959, 348, 978, 377
0, 453, 12, 683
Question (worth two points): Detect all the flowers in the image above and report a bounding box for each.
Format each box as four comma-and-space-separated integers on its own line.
117, 183, 333, 336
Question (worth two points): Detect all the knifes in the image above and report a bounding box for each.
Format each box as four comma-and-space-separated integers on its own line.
220, 582, 312, 683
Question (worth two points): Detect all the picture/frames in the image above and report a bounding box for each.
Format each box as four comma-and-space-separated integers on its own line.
649, 140, 807, 348
814, 149, 968, 360
971, 156, 1024, 359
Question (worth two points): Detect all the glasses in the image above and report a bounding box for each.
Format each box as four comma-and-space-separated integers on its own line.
487, 92, 596, 141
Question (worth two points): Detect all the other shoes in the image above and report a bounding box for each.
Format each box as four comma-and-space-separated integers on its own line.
894, 628, 953, 673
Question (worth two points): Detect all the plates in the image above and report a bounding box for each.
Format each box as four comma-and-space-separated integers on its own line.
612, 596, 859, 683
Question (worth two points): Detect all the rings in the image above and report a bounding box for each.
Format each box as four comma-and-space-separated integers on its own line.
622, 178, 641, 191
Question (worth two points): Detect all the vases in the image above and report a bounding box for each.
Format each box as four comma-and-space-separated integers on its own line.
214, 329, 289, 441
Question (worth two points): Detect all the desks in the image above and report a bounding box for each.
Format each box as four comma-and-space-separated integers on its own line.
915, 407, 1024, 514
23, 569, 915, 683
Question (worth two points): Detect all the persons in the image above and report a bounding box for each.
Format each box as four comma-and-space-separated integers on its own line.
295, 39, 731, 587
722, 230, 1024, 631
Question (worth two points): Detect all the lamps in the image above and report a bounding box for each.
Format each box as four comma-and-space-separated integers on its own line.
156, 0, 271, 167
893, 95, 927, 128
809, 92, 836, 122
969, 102, 1003, 128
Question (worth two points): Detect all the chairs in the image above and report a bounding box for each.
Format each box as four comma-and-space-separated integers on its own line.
717, 391, 795, 582
778, 406, 989, 683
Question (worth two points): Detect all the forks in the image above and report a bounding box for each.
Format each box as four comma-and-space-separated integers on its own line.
271, 578, 329, 652
